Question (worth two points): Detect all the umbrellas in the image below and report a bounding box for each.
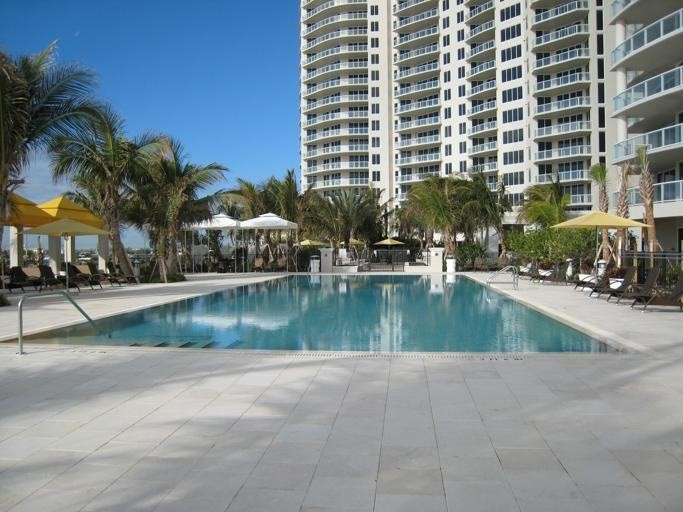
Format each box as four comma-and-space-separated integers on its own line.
16, 216, 111, 292
339, 237, 365, 249
374, 236, 406, 256
549, 211, 652, 285
295, 239, 326, 255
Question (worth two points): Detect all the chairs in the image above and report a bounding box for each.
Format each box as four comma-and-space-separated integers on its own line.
0, 259, 139, 294
518, 261, 683, 312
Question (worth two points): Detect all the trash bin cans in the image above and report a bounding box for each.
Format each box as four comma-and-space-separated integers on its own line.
310, 255, 320, 273
446, 255, 457, 273
